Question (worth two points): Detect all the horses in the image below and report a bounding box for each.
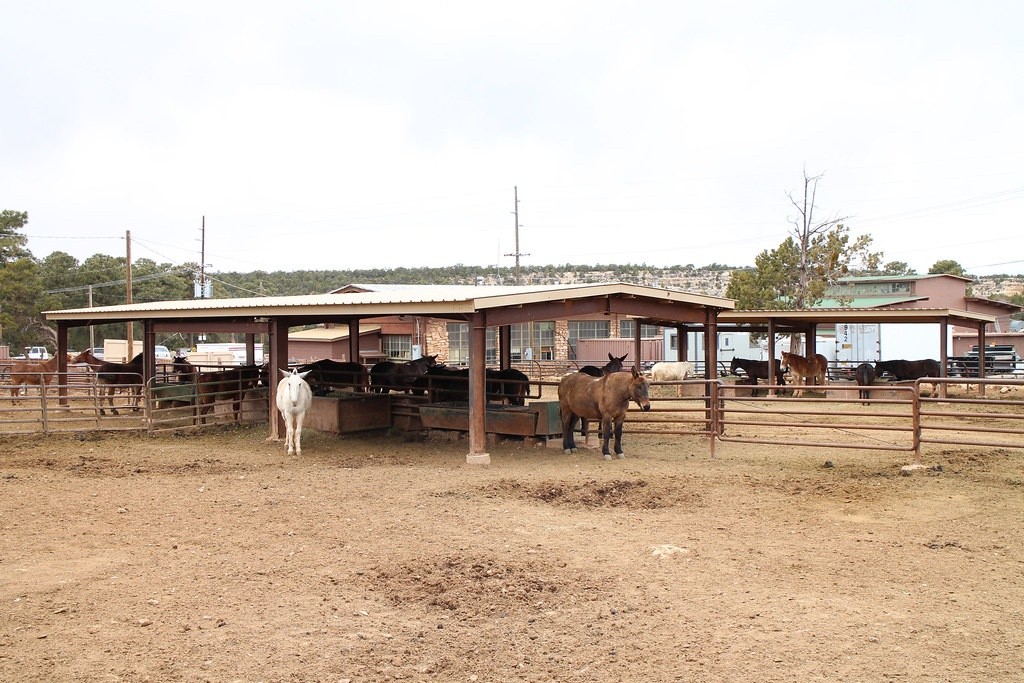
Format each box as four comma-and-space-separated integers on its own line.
369, 354, 439, 394
171, 356, 197, 382
72, 350, 143, 416
780, 351, 830, 398
190, 363, 265, 436
558, 365, 651, 460
2, 347, 73, 406
577, 352, 629, 438
873, 359, 940, 398
730, 356, 788, 397
299, 358, 370, 393
276, 367, 313, 457
412, 361, 530, 407
855, 364, 874, 406
651, 360, 697, 397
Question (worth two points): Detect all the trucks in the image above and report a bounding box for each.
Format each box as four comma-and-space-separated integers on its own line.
835, 324, 956, 372
12, 346, 49, 361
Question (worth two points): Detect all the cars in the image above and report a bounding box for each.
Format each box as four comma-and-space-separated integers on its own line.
84, 348, 104, 360
155, 345, 170, 359
